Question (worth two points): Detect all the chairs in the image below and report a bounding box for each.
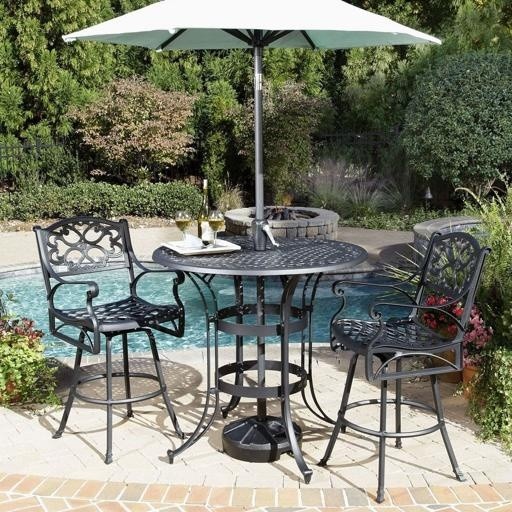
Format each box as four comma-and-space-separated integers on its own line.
317, 231, 492, 503
33, 219, 187, 464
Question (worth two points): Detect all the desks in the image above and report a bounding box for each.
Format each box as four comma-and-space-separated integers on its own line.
152, 235, 368, 483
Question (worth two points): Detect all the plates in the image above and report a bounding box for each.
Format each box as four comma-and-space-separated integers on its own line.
162, 238, 241, 255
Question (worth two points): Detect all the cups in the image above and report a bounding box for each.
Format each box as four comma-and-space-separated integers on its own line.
176, 211, 190, 246
208, 211, 225, 248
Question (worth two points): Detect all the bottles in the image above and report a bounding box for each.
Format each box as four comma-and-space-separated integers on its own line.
198, 179, 212, 239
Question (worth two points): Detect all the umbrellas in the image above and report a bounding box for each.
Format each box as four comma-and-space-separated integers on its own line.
59, 0, 441, 252
